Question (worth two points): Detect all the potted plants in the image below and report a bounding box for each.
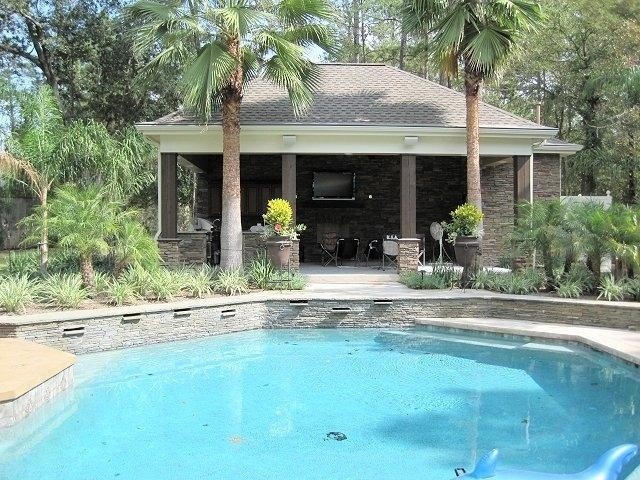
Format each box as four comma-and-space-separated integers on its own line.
262, 197, 294, 270
448, 202, 484, 268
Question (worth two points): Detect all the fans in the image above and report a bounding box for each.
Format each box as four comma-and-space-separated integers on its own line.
429, 222, 444, 263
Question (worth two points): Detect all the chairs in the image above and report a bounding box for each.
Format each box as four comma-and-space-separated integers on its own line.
320, 232, 399, 275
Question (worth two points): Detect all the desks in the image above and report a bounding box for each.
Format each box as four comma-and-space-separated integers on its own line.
416, 234, 425, 266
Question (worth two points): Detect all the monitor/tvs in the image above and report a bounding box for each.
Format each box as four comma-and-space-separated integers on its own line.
314, 173, 353, 198
